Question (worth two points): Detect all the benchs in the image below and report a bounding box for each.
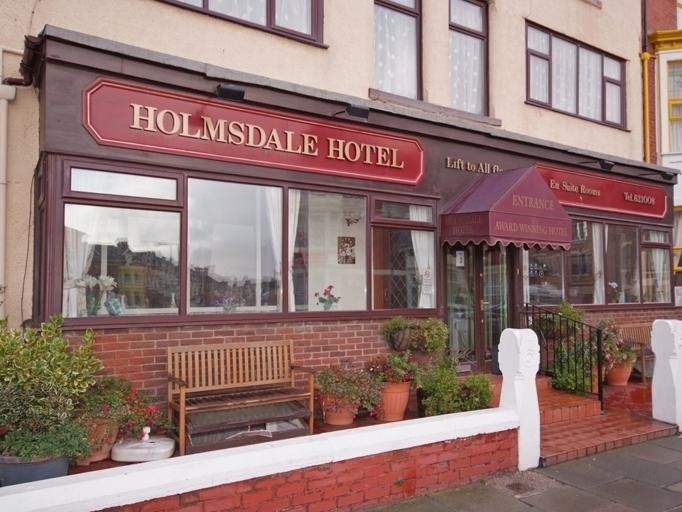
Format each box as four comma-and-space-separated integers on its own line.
611, 322, 656, 385
166, 339, 317, 456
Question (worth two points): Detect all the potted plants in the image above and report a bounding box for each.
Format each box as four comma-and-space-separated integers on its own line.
528, 302, 637, 395
0, 313, 119, 487
314, 349, 421, 425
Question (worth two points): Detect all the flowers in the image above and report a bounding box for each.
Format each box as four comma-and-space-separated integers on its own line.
84, 376, 177, 457
77, 275, 118, 315
313, 286, 341, 310
